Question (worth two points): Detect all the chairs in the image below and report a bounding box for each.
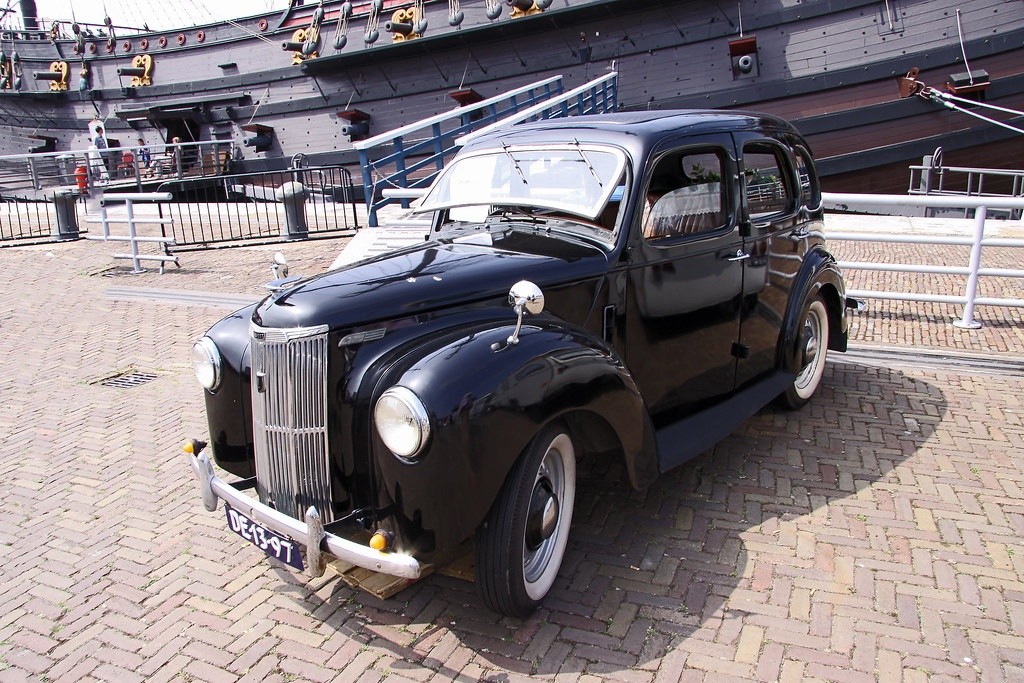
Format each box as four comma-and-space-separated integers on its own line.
642, 182, 721, 239
117, 152, 133, 177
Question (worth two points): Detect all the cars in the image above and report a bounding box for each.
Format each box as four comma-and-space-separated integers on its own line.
182, 107, 860, 620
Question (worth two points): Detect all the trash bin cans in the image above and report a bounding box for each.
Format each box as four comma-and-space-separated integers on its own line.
48, 190, 82, 240
276, 181, 310, 241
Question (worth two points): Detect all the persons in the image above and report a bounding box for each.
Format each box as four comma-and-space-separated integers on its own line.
137, 139, 154, 178
172, 137, 183, 164
95, 127, 109, 171
